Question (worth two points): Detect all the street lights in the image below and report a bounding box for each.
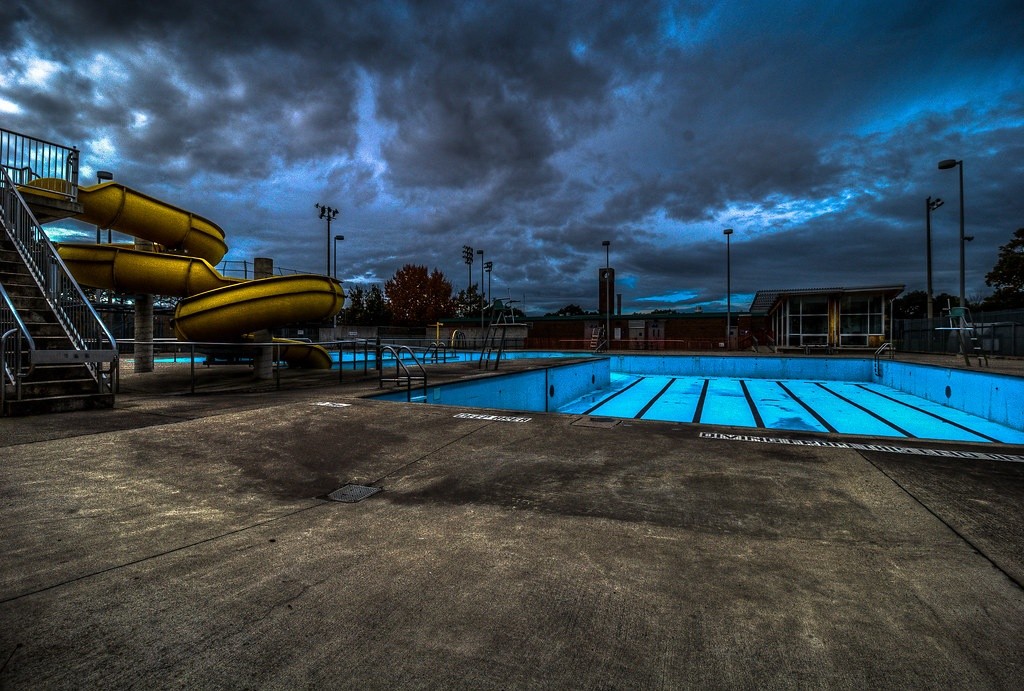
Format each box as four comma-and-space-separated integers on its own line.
477, 249, 484, 350
601, 241, 610, 350
937, 159, 973, 356
333, 235, 344, 328
314, 202, 339, 276
461, 245, 474, 320
926, 196, 945, 354
724, 229, 733, 352
